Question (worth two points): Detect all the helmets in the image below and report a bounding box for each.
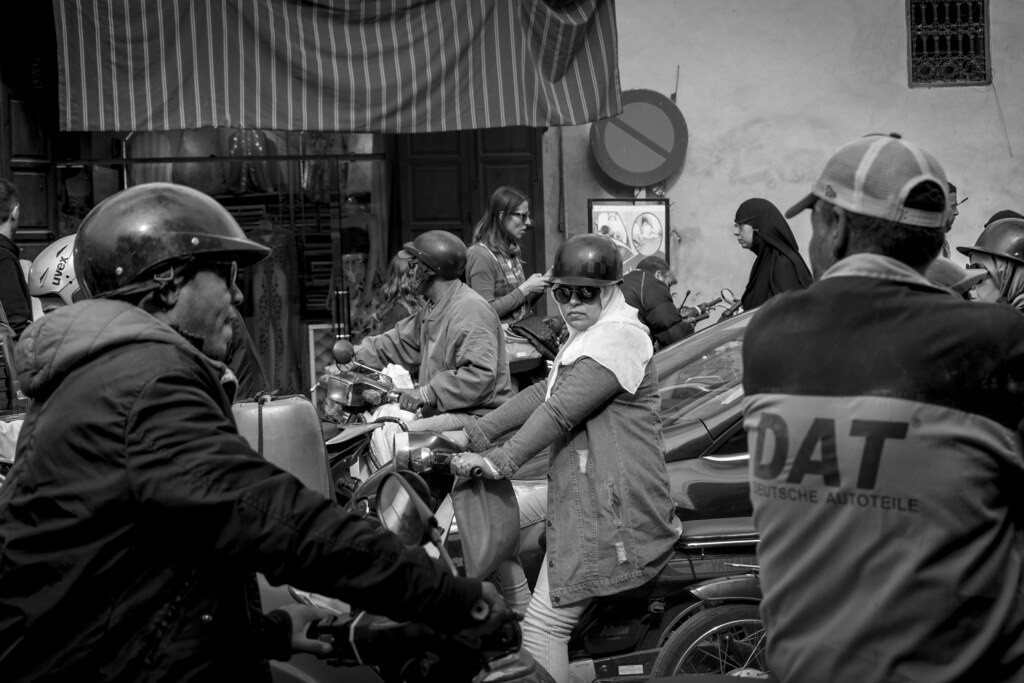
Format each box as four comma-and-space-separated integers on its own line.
956, 218, 1024, 263
403, 230, 468, 281
72, 182, 272, 299
544, 234, 624, 286
27, 233, 88, 304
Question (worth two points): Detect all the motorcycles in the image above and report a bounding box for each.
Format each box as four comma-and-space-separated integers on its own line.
301, 288, 751, 424
286, 416, 771, 682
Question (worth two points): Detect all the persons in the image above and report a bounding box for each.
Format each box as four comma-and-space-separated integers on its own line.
0, 182, 508, 683
321, 186, 698, 683
734, 133, 1024, 683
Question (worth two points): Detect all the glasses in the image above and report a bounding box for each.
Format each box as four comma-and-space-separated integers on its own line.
509, 211, 531, 225
553, 286, 600, 304
408, 261, 419, 270
966, 264, 983, 285
209, 260, 238, 289
734, 216, 754, 230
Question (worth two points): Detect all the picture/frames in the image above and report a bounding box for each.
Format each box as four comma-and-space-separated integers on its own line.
309, 325, 347, 410
587, 197, 671, 268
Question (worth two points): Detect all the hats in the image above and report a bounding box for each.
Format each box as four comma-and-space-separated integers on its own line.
785, 132, 951, 228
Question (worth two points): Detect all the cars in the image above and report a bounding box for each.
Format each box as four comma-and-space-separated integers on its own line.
437, 304, 754, 591
